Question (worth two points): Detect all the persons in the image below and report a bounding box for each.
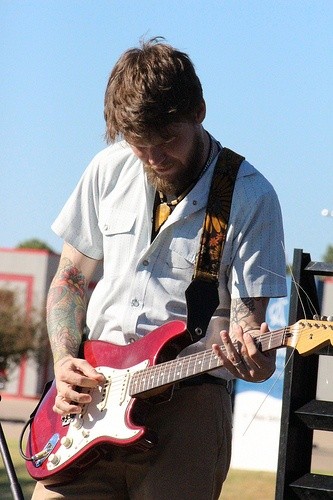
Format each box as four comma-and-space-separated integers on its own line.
27, 36, 287, 500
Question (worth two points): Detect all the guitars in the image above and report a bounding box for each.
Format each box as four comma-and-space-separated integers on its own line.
18, 313, 333, 489
